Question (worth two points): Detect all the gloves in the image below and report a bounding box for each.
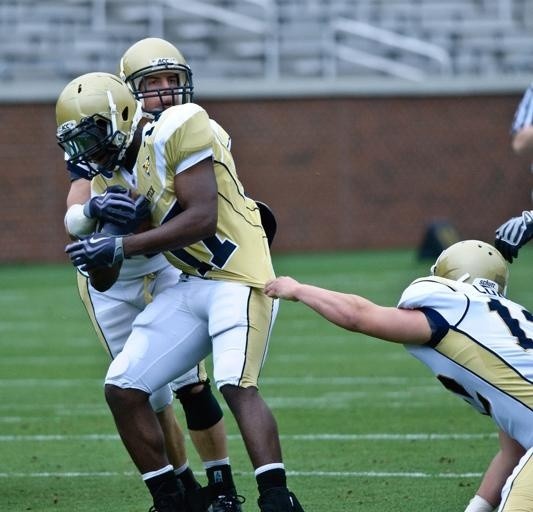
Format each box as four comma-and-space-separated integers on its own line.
65, 232, 124, 271
83, 185, 139, 226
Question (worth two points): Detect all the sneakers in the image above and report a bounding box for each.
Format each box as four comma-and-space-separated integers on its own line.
148, 486, 304, 511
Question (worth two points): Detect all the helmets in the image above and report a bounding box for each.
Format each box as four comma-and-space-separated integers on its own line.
430, 239, 507, 296
55, 72, 139, 177
120, 38, 195, 116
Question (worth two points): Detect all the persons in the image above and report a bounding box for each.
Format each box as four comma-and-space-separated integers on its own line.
510, 83, 533, 162
54, 70, 246, 511
264, 239, 533, 511
64, 38, 304, 512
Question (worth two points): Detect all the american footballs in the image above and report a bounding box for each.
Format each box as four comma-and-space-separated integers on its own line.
86, 199, 126, 292
